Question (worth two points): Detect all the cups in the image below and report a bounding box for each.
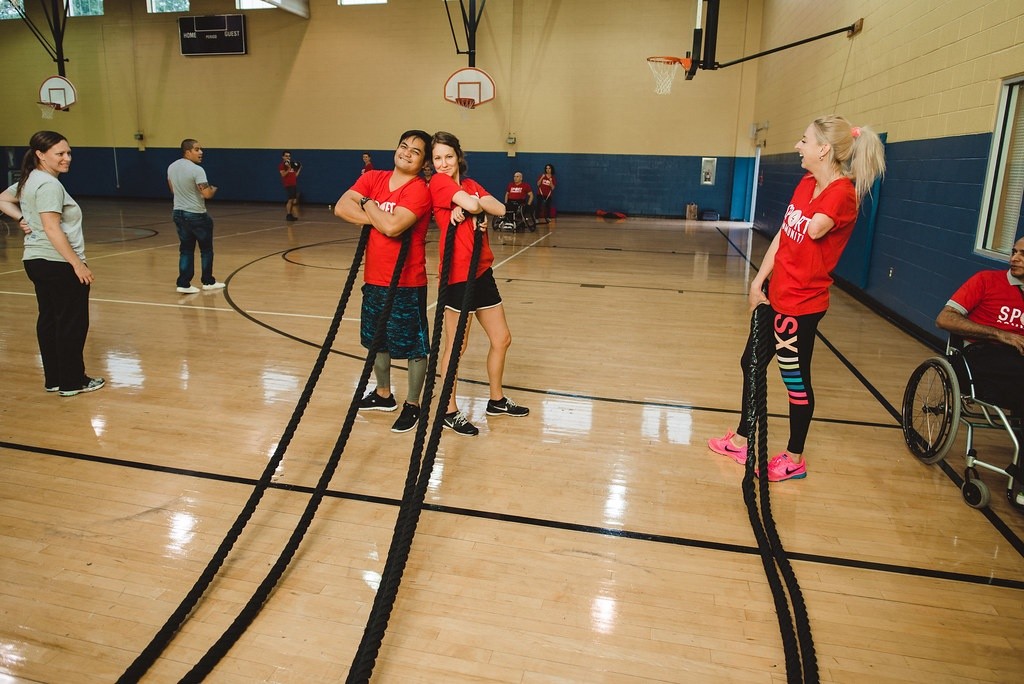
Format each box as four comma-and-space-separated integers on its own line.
329, 205, 331, 208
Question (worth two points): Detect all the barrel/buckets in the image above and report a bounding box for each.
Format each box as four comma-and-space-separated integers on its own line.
686, 202, 698, 219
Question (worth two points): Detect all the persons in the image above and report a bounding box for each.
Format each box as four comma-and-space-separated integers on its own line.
935, 235, 1024, 504
168, 139, 226, 292
423, 166, 432, 186
334, 130, 432, 431
280, 151, 302, 220
708, 114, 885, 481
430, 132, 528, 436
505, 172, 534, 221
535, 164, 556, 223
361, 153, 374, 174
0, 131, 106, 396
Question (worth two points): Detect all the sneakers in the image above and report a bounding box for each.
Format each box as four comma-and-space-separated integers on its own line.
442, 411, 478, 436
391, 401, 421, 433
202, 282, 226, 291
45, 386, 59, 392
709, 430, 748, 465
57, 377, 106, 397
755, 452, 807, 482
486, 395, 529, 416
177, 285, 200, 293
358, 389, 397, 412
286, 213, 298, 220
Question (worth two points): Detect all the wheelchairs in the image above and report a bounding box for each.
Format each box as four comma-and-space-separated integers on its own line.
901, 333, 1024, 509
491, 200, 537, 233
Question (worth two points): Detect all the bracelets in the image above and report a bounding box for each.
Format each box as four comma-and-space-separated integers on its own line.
18, 216, 23, 222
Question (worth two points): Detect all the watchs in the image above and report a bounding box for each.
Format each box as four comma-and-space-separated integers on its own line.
361, 197, 372, 210
